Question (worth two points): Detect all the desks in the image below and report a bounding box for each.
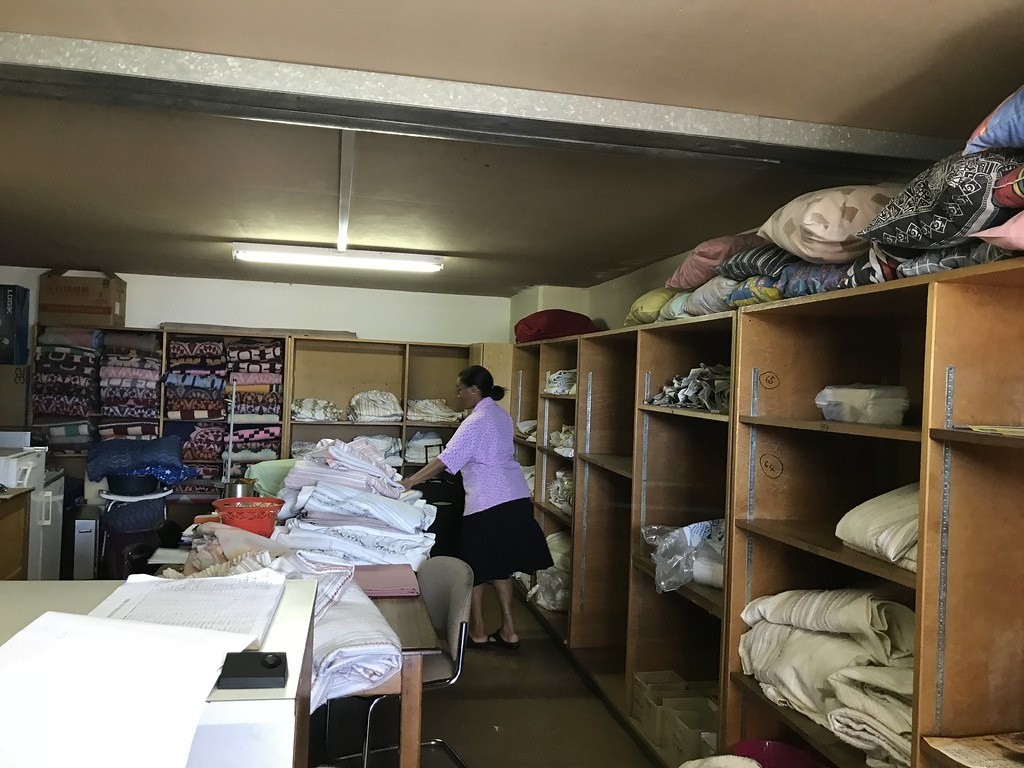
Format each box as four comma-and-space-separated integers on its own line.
156, 544, 444, 768
0, 581, 319, 766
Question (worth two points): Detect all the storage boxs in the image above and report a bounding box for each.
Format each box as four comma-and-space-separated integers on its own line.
38, 254, 128, 328
633, 670, 688, 723
641, 680, 719, 747
1, 365, 30, 430
0, 284, 30, 364
661, 694, 720, 768
213, 497, 286, 539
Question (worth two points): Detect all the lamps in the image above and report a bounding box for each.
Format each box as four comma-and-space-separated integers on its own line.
231, 242, 445, 276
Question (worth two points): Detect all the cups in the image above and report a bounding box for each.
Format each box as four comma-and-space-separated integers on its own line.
224, 483, 254, 498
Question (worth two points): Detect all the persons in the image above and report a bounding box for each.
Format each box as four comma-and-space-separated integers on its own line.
399, 364, 556, 651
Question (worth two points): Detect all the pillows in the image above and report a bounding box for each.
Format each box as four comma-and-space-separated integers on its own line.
622, 83, 1024, 327
514, 309, 598, 343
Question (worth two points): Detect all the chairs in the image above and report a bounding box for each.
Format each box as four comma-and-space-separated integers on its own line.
325, 555, 475, 768
156, 521, 185, 549
122, 542, 164, 576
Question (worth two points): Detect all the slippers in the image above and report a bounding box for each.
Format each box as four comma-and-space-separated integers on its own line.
465, 629, 520, 650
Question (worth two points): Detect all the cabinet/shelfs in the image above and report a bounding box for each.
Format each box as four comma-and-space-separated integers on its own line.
567, 310, 736, 767
286, 335, 473, 482
721, 257, 1024, 768
27, 322, 289, 532
44, 467, 65, 581
1, 446, 52, 581
511, 334, 580, 648
470, 341, 513, 417
1, 486, 37, 580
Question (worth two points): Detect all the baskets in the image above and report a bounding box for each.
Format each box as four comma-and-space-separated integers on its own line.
211, 497, 286, 539
